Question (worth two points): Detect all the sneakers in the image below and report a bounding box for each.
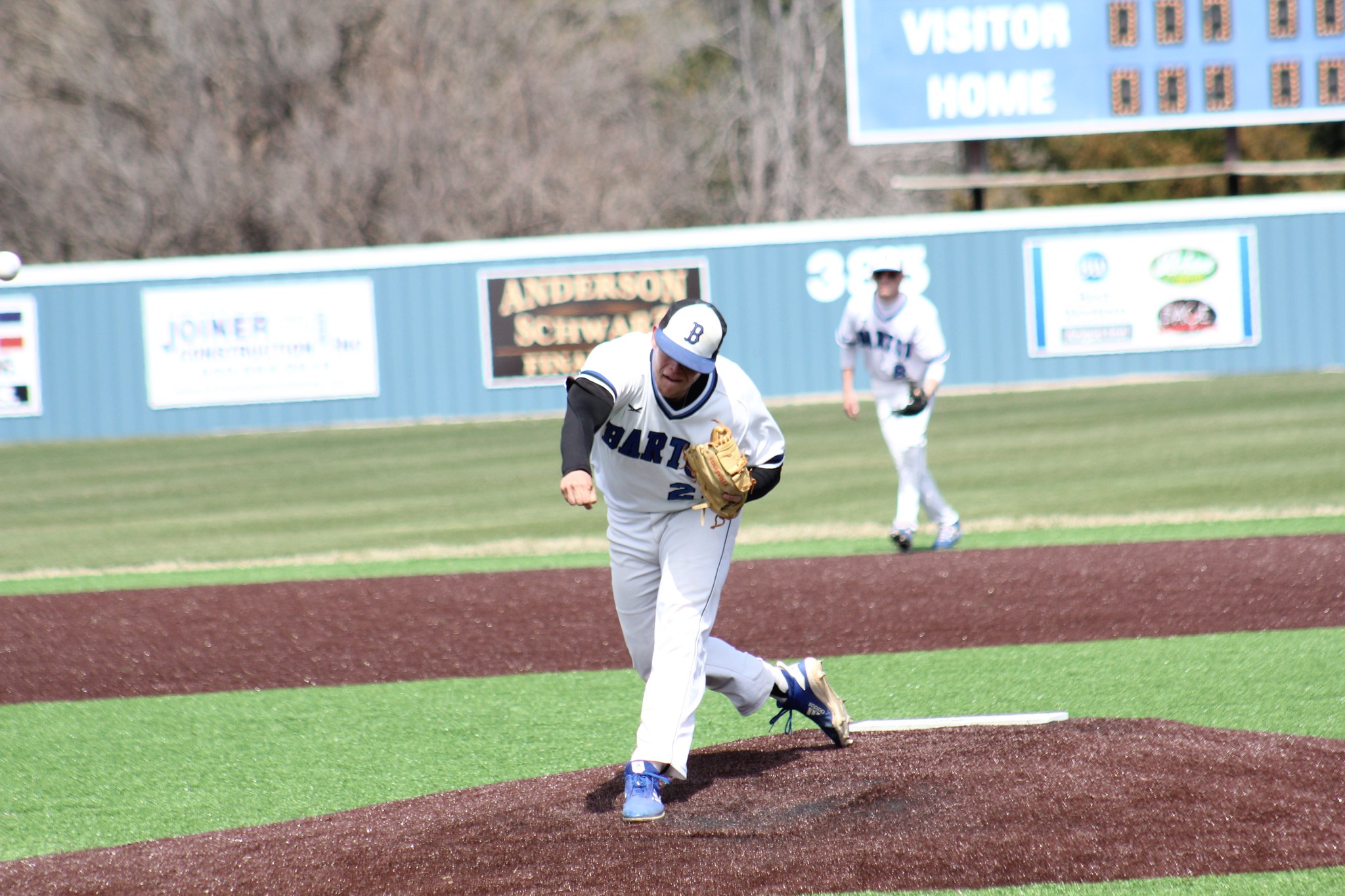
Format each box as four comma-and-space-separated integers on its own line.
622, 761, 671, 820
891, 529, 912, 553
930, 522, 962, 550
767, 657, 854, 748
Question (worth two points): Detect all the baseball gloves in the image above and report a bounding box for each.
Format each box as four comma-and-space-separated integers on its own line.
890, 377, 928, 417
683, 426, 753, 520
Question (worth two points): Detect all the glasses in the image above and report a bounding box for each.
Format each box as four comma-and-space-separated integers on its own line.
873, 271, 898, 281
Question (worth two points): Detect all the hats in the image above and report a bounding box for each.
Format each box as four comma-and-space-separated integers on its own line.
871, 246, 902, 274
654, 298, 727, 374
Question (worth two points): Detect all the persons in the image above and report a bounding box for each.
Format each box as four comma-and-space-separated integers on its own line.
559, 298, 853, 823
835, 257, 963, 552
